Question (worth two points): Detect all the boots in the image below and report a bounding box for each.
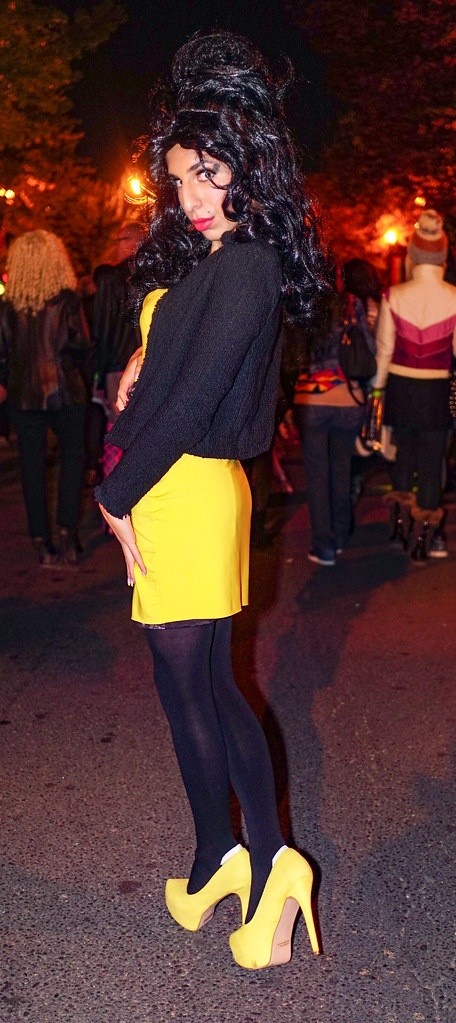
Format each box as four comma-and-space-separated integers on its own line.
383, 492, 414, 550
407, 507, 443, 566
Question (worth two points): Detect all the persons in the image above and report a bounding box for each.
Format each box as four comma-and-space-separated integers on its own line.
0, 210, 456, 568
96, 33, 322, 972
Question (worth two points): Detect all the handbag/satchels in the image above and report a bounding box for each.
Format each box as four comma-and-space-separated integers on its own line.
340, 293, 377, 380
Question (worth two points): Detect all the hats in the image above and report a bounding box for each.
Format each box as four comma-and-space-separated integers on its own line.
408, 209, 448, 265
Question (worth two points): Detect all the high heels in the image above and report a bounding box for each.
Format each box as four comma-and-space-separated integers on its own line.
228, 847, 320, 970
165, 849, 252, 931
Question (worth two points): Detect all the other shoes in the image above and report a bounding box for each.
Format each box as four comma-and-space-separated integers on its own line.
429, 530, 448, 558
59, 534, 81, 563
308, 551, 335, 567
33, 540, 58, 567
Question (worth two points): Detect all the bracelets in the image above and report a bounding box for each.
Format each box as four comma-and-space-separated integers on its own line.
368, 383, 387, 398
